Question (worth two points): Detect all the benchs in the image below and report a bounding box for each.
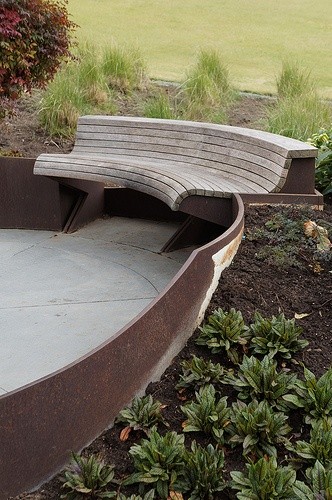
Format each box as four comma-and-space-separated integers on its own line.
32, 113, 323, 255
